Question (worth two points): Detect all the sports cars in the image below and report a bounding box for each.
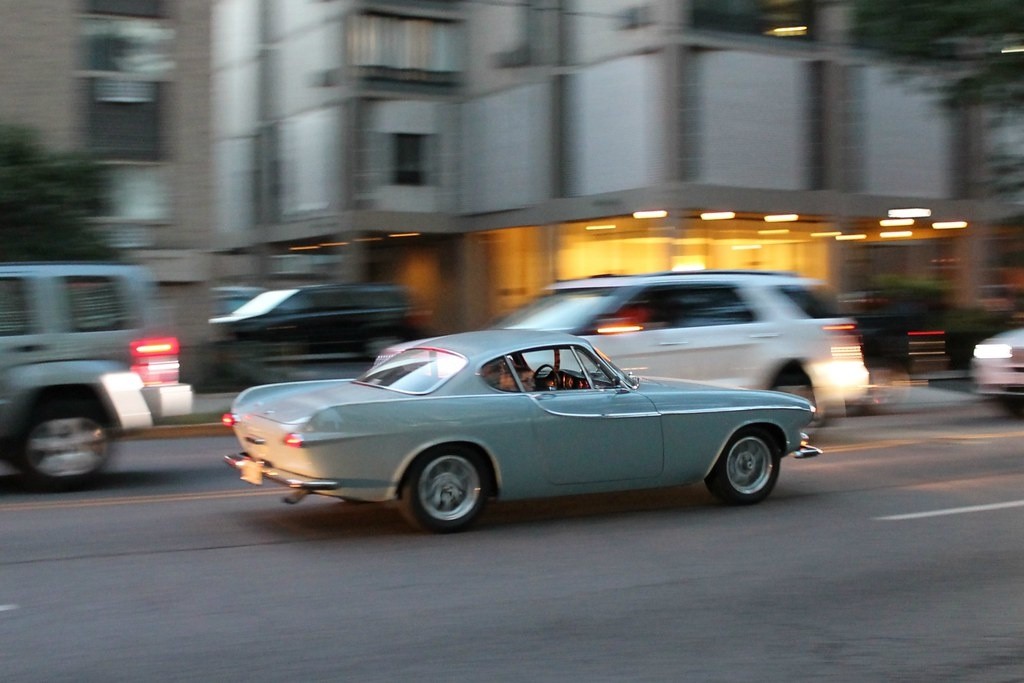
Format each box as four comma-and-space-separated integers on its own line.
221, 329, 823, 535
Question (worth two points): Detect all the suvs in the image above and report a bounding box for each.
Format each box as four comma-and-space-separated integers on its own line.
369, 268, 871, 420
207, 282, 406, 364
0, 260, 195, 493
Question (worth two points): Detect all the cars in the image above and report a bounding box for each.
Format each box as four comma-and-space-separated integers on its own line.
967, 328, 1024, 419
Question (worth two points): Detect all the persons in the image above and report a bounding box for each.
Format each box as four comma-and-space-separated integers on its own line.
513, 365, 535, 391
